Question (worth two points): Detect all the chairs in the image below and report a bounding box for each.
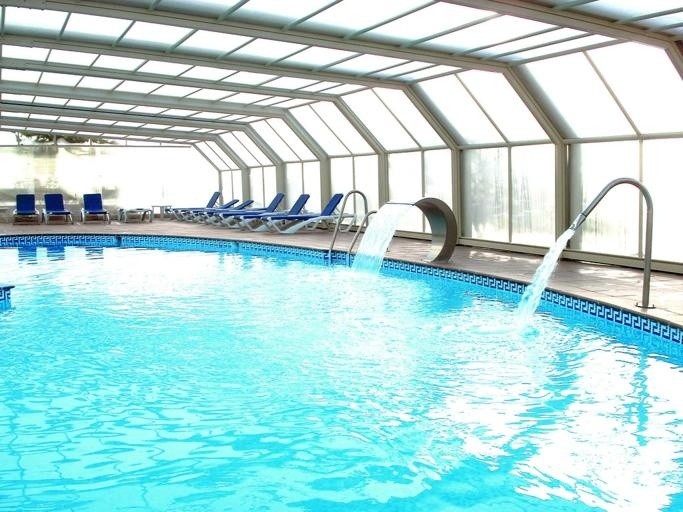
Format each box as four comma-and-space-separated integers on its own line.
11, 192, 111, 226
165, 191, 354, 235
